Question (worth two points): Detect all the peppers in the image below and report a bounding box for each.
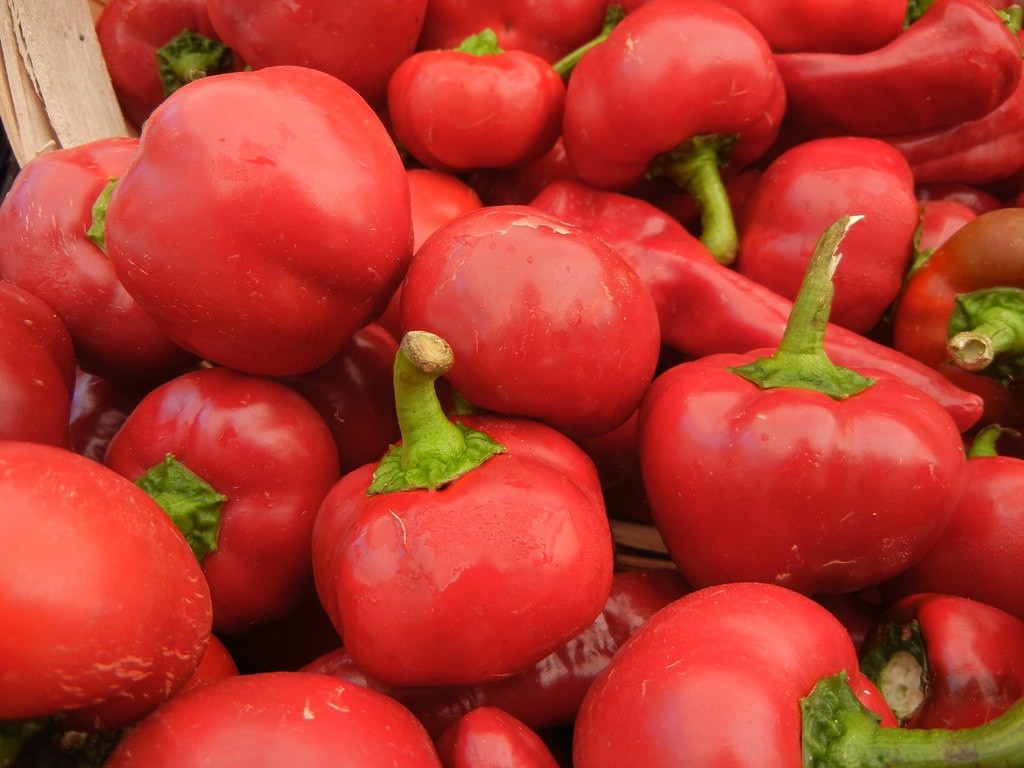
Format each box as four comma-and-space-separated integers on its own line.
0, 0, 1024, 768
